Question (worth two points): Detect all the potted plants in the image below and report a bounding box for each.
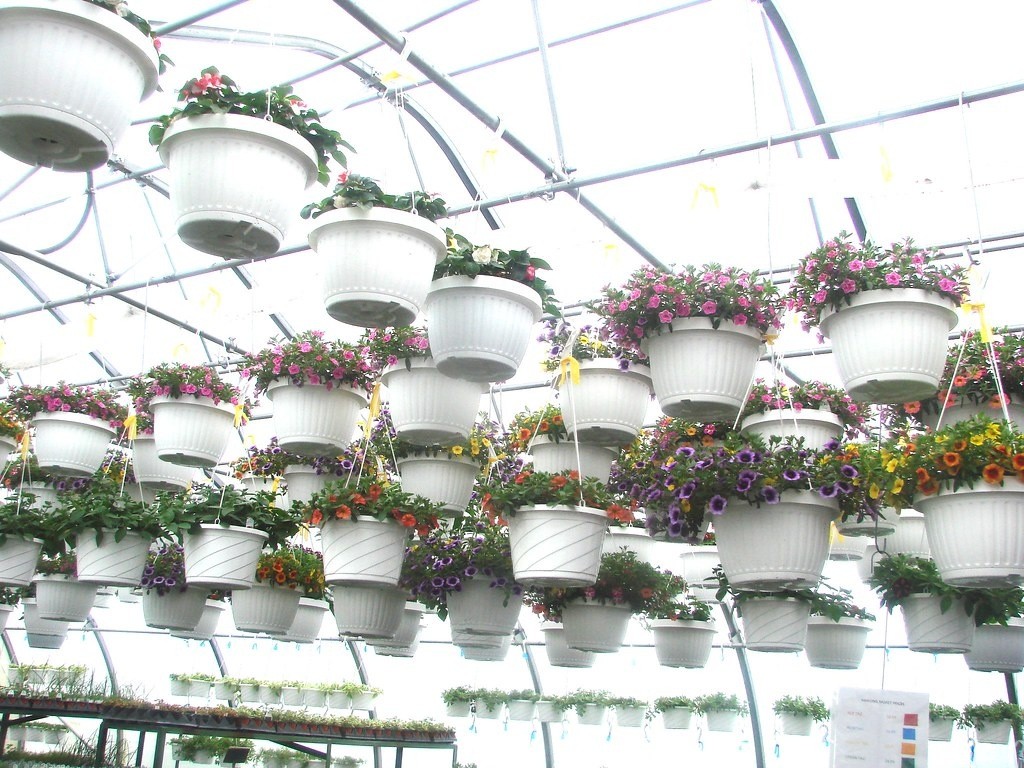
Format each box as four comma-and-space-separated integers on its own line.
0, 671, 1024, 768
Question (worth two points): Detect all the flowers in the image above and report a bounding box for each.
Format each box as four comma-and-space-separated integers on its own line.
0, 323, 1024, 668
162, 68, 974, 325
93, 0, 173, 86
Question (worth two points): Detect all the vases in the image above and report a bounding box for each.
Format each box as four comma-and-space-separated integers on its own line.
0, 0, 160, 173
163, 117, 958, 414
0, 358, 1024, 669
